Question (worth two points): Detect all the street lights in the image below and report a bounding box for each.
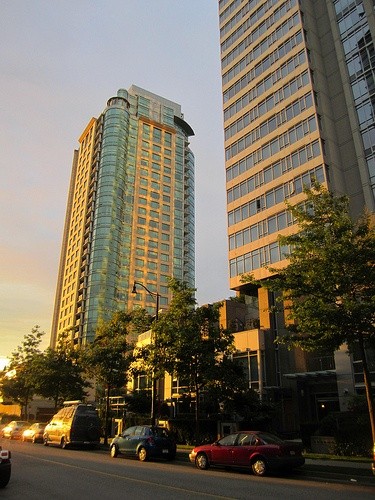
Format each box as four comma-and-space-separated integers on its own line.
131, 280, 161, 422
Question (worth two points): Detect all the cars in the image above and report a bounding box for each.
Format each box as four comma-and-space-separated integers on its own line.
22, 422, 49, 442
110, 425, 175, 462
2, 421, 33, 440
189, 429, 304, 475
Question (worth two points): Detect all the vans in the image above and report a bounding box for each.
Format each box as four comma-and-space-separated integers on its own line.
43, 405, 102, 451
